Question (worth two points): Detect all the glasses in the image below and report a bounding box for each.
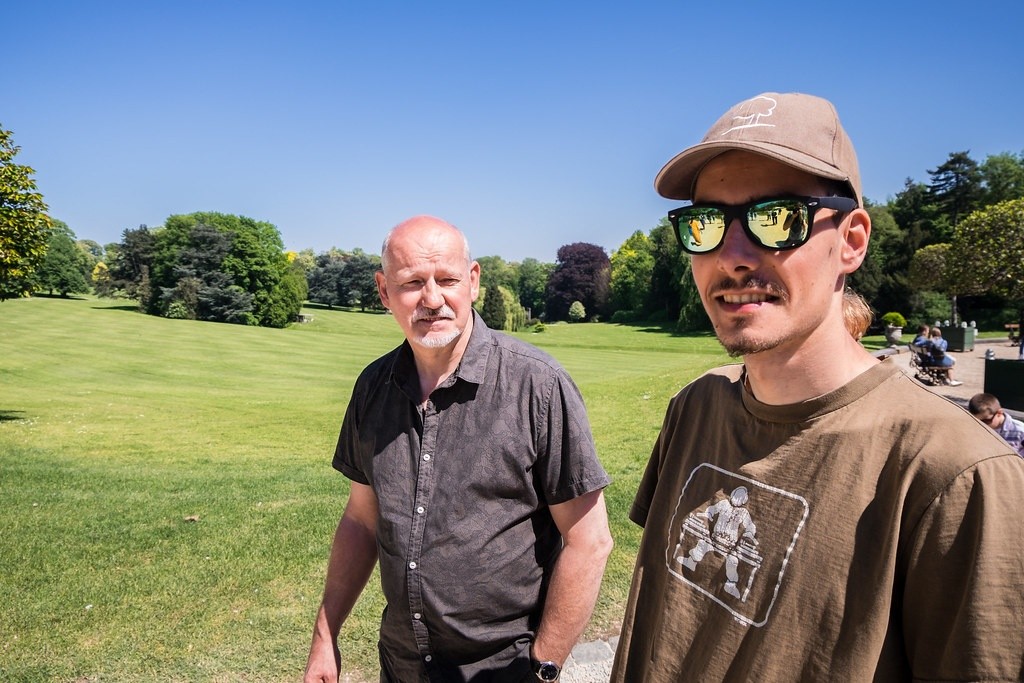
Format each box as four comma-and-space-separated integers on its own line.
668, 195, 858, 255
982, 412, 997, 425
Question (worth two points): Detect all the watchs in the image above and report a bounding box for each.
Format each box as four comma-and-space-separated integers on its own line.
529, 642, 564, 683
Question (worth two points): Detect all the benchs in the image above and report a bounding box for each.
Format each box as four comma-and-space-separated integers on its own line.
1005, 323, 1023, 347
907, 342, 954, 386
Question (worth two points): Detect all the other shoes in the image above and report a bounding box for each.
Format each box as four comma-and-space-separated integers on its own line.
951, 382, 963, 386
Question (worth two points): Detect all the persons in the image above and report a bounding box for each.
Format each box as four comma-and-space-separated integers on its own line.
302, 214, 614, 683
684, 202, 807, 250
611, 89, 1024, 682
911, 323, 964, 386
965, 393, 1023, 457
837, 288, 873, 343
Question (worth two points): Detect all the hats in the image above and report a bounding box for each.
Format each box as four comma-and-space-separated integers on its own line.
654, 93, 863, 208
